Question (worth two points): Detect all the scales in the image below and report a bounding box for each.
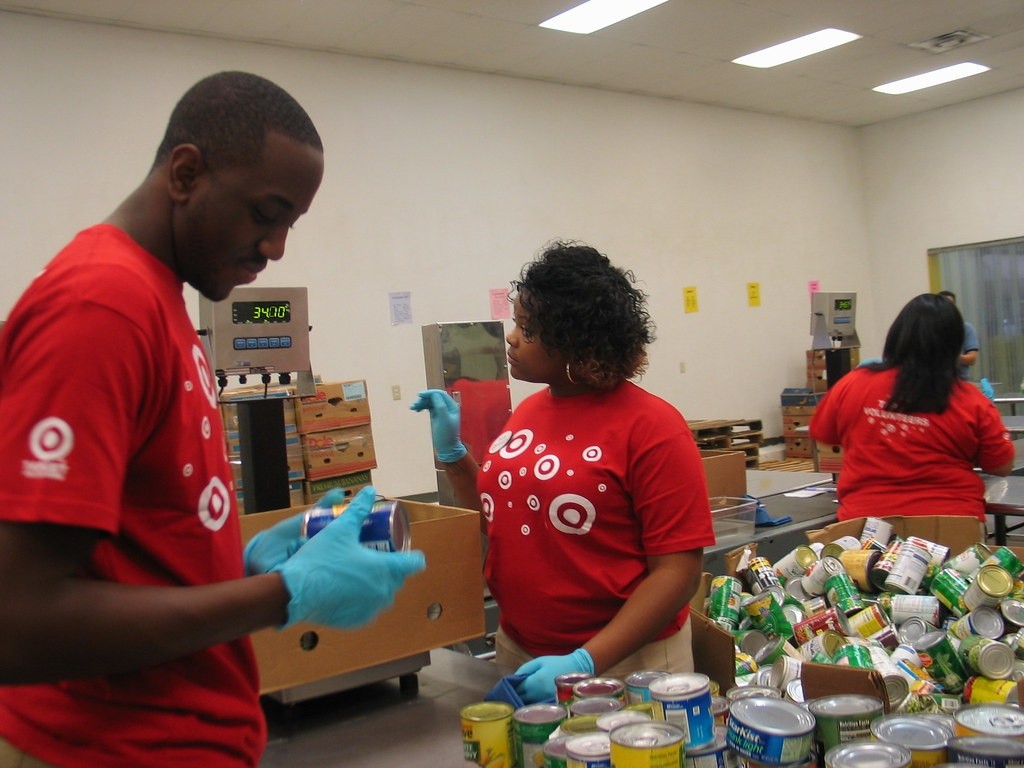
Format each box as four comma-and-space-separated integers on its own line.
809, 290, 863, 485
190, 284, 432, 735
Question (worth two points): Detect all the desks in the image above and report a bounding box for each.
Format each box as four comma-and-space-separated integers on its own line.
993, 396, 1024, 416
1000, 416, 1024, 440
1008, 438, 1024, 471
966, 474, 1024, 546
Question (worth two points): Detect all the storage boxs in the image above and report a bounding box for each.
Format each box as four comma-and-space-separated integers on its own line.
217, 382, 298, 442
232, 492, 487, 694
780, 346, 861, 472
305, 469, 374, 505
225, 432, 306, 489
235, 482, 306, 516
300, 425, 378, 482
293, 375, 376, 436
691, 513, 1024, 723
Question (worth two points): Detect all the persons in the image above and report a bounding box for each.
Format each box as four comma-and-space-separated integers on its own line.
409, 238, 715, 708
939, 291, 979, 381
0, 70, 427, 768
808, 293, 1015, 552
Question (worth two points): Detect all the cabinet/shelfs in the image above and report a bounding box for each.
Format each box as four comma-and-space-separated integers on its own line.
685, 417, 765, 469
